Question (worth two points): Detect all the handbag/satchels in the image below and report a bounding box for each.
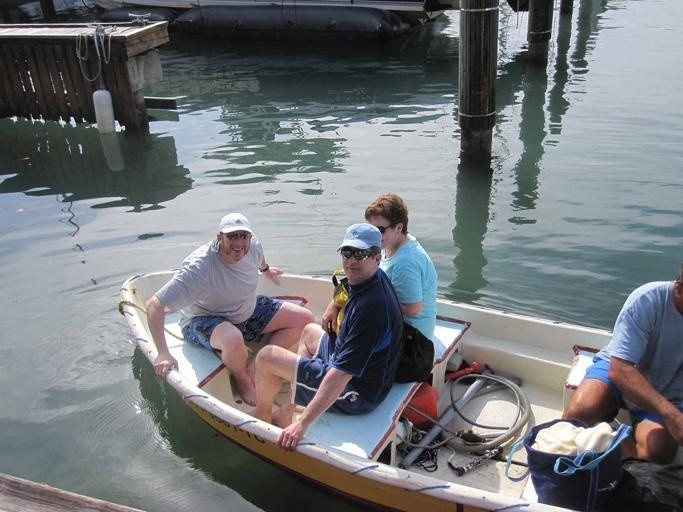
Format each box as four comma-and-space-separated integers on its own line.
505, 419, 632, 512
395, 320, 435, 383
332, 269, 350, 336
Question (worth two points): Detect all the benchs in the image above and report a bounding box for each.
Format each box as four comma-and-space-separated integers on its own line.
291, 313, 473, 468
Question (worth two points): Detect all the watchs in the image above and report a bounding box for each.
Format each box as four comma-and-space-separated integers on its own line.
259, 263, 269, 272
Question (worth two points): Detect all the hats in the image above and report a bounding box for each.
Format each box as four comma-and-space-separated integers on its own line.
219, 213, 252, 233
336, 223, 382, 252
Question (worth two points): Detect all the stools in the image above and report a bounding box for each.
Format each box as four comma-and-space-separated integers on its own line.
164, 294, 309, 407
562, 344, 633, 430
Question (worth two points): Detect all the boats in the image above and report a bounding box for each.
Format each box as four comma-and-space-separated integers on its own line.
114, 264, 683, 511
90, 0, 457, 36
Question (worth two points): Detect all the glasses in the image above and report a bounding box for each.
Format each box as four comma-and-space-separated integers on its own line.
376, 224, 393, 233
341, 245, 376, 262
226, 231, 251, 240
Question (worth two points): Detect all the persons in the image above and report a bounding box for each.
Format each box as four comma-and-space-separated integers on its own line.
243, 223, 403, 451
364, 193, 437, 343
561, 264, 683, 463
147, 212, 315, 407
